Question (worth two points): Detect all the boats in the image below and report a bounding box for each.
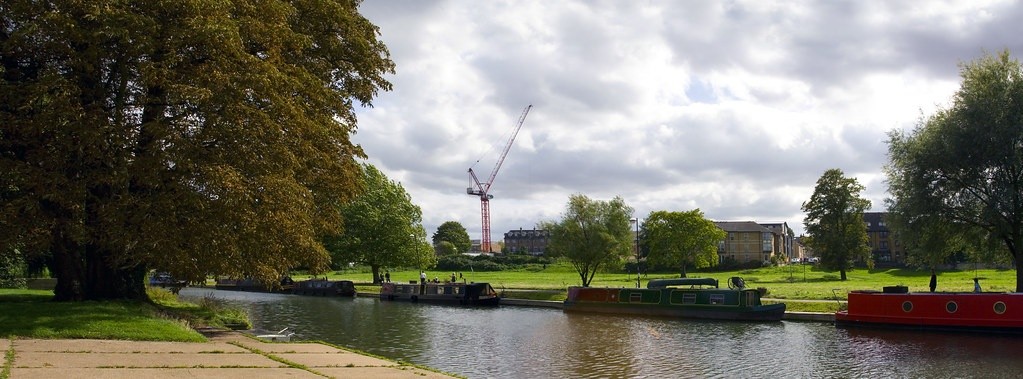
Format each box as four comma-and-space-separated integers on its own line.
214, 277, 241, 291
293, 280, 355, 297
236, 274, 297, 295
562, 278, 788, 321
378, 280, 503, 308
148, 269, 189, 286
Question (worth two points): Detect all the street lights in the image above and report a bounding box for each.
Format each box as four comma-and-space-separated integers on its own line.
629, 217, 640, 288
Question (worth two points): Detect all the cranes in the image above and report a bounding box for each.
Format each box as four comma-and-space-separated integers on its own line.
465, 102, 535, 253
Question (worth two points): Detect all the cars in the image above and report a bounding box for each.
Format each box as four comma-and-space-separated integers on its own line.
834, 285, 1023, 334
808, 257, 819, 263
790, 257, 800, 265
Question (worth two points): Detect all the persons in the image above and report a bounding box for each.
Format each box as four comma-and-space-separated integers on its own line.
973, 278, 981, 292
929, 268, 937, 293
420, 271, 464, 282
379, 270, 391, 283
